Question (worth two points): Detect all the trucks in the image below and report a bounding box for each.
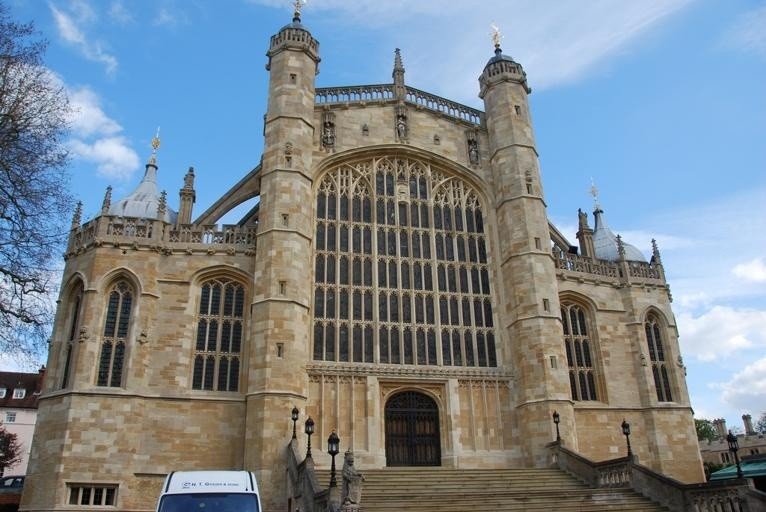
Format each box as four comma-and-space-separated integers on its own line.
156, 470, 262, 511
